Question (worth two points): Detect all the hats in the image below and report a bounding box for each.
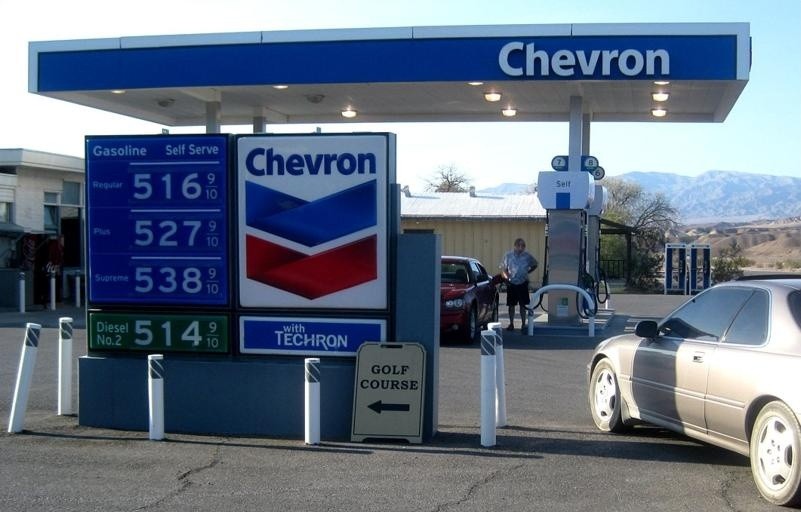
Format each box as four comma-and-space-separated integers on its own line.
588, 273, 801, 506
439, 255, 501, 342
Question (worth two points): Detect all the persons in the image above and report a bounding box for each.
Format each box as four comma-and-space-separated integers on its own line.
39, 241, 61, 307
500, 239, 538, 332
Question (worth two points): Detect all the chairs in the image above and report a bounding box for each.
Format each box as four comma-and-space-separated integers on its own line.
506, 323, 515, 331
521, 324, 527, 332
